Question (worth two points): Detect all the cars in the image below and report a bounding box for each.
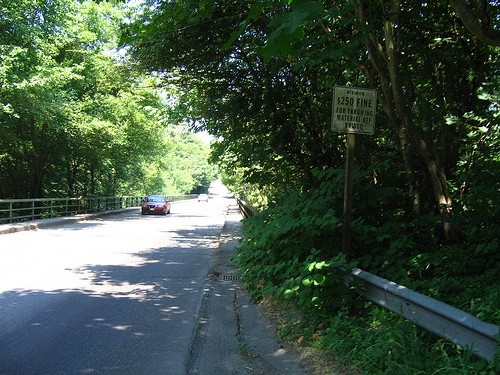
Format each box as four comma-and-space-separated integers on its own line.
197, 194, 208, 202
141, 194, 171, 216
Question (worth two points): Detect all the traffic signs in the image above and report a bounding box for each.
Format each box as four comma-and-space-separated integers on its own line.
331, 84, 377, 136
